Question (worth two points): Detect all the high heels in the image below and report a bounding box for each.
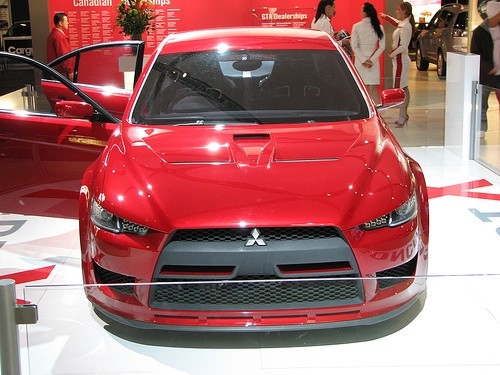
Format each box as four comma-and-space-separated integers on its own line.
394, 114, 409, 128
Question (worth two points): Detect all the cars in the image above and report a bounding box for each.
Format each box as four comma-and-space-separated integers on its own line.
0, 25, 430, 333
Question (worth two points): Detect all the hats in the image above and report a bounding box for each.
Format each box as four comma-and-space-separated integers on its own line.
485, 1, 500, 19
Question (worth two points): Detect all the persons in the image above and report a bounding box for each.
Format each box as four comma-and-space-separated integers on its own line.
47, 13, 74, 83
380, 2, 415, 129
311, 0, 349, 47
471, 1, 500, 138
350, 2, 386, 105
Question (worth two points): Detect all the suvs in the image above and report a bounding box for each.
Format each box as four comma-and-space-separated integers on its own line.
407, 1, 469, 77
